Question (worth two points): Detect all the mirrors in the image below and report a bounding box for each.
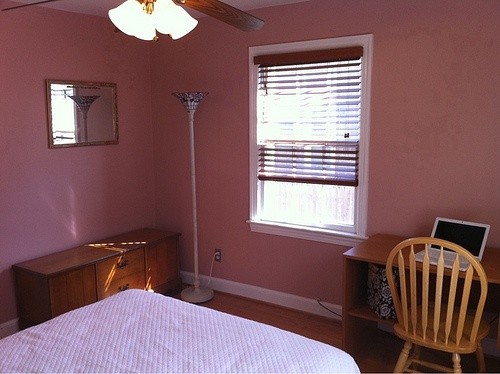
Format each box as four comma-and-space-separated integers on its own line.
46, 79, 119, 150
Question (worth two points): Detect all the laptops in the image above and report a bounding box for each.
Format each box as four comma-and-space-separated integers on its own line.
415, 217, 490, 271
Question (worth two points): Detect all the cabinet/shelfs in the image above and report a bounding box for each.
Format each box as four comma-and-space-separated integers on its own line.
12, 227, 182, 332
341, 233, 500, 374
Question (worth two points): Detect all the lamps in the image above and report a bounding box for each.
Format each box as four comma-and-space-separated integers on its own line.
69, 95, 100, 141
172, 90, 214, 304
108, 0, 198, 41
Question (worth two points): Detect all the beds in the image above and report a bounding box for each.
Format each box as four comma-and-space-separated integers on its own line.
0, 289, 362, 374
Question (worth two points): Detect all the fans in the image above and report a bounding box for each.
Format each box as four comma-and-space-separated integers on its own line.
114, 0, 266, 55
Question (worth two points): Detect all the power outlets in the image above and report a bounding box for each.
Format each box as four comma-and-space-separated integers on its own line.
214, 249, 220, 263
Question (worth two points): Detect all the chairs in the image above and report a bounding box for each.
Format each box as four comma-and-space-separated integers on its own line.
386, 237, 491, 374
